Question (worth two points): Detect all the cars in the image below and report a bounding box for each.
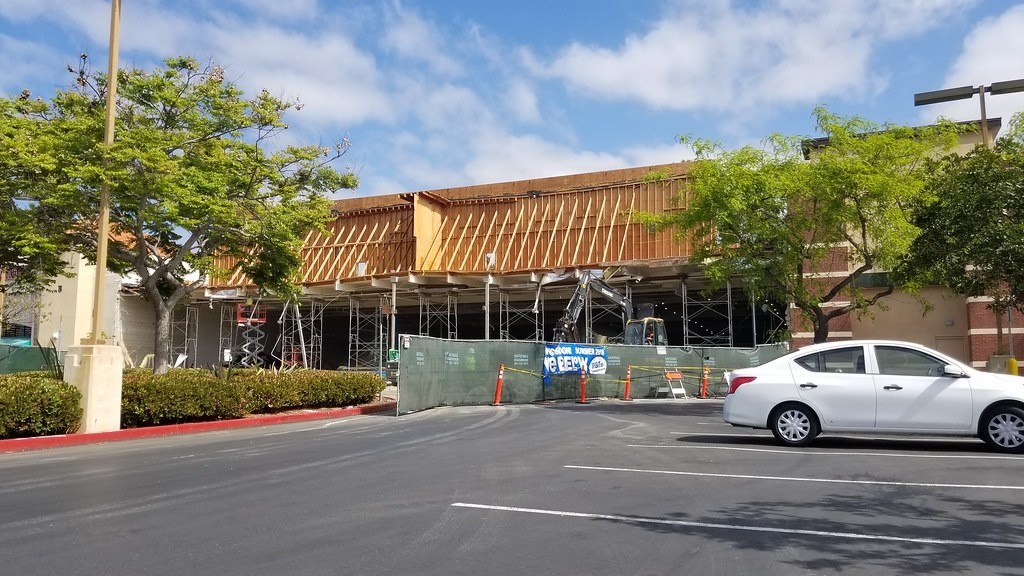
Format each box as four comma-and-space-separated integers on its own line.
721, 340, 1024, 455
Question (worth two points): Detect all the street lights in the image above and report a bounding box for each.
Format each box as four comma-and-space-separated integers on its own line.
914, 78, 1024, 353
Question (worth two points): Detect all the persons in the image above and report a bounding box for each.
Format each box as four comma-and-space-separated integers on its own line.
645, 328, 654, 342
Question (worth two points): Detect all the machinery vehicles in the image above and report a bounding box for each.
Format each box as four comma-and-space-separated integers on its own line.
551, 270, 669, 345
